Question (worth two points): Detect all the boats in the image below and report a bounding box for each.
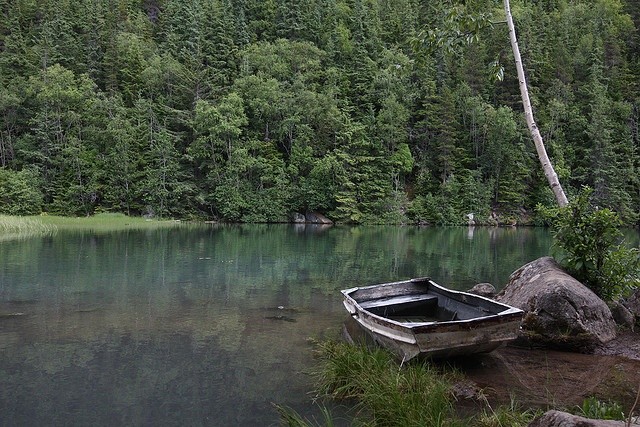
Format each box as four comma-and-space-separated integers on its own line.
341, 277, 525, 363
